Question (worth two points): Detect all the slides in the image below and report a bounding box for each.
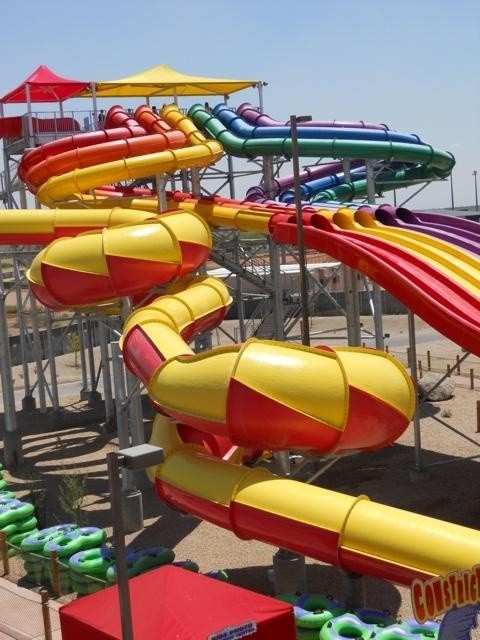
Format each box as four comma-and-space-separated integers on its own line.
274, 206, 479, 361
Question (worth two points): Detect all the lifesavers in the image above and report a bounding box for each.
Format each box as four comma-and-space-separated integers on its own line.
0, 463, 228, 595
276, 594, 442, 639
